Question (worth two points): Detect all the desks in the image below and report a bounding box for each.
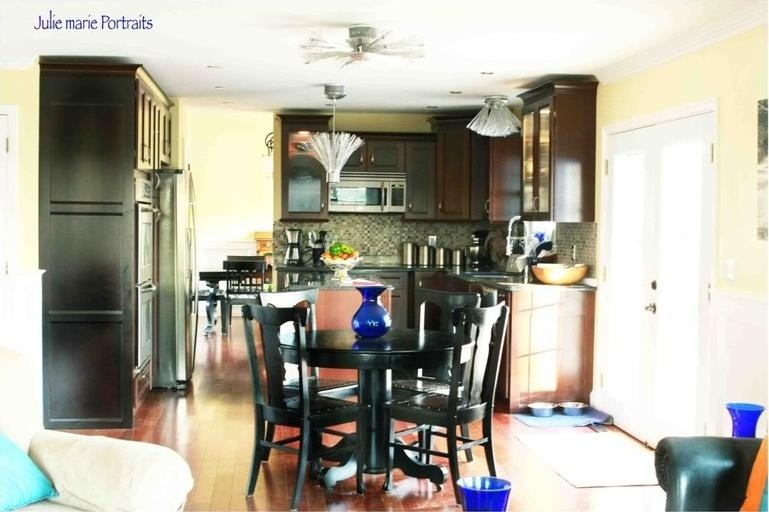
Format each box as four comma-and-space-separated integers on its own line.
196, 270, 270, 333
275, 327, 475, 474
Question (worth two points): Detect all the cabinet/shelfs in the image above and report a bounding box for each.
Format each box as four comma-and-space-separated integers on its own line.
277, 267, 597, 414
39, 62, 175, 429
276, 81, 599, 224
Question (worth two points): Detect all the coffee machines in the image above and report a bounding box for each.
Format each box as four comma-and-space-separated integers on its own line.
285, 272, 299, 286
284, 229, 302, 265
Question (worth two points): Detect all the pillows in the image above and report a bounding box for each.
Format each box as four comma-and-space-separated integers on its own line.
1, 431, 60, 512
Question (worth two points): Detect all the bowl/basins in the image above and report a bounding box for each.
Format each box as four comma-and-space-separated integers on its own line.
559, 401, 589, 416
531, 262, 589, 285
528, 402, 558, 417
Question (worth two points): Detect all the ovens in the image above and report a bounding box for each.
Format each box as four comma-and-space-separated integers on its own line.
133, 176, 158, 373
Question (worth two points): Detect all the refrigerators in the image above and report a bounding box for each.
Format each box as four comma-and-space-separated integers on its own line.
155, 167, 201, 391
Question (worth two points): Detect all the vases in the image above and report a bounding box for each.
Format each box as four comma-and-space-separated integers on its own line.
727, 403, 765, 438
456, 477, 511, 511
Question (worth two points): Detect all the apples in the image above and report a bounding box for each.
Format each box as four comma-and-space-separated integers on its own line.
323, 241, 358, 259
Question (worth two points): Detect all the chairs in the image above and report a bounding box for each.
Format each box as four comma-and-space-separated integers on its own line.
239, 304, 365, 512
227, 256, 266, 325
260, 300, 358, 473
393, 285, 481, 461
382, 300, 510, 504
222, 262, 264, 333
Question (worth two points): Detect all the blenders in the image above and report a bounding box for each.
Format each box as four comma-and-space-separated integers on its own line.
308, 230, 328, 267
305, 268, 327, 287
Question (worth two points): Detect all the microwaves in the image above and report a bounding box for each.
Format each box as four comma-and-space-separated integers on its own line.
327, 171, 406, 213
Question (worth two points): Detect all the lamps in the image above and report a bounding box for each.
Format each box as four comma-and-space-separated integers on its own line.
301, 26, 424, 78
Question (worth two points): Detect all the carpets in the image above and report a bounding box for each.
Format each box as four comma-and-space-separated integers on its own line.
515, 431, 661, 487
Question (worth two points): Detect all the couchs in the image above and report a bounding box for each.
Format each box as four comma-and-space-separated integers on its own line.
1, 430, 194, 512
654, 435, 768, 512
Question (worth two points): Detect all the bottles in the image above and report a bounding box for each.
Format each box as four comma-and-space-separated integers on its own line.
468, 235, 487, 268
402, 242, 464, 269
